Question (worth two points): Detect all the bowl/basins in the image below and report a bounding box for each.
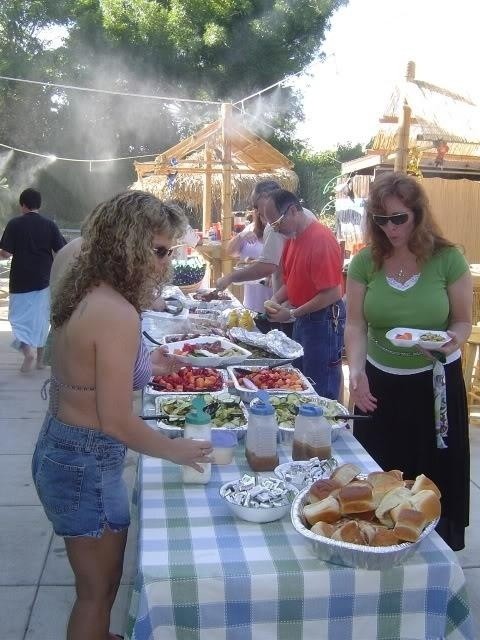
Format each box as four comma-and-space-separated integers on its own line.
219, 477, 299, 524
273, 461, 311, 480
291, 473, 440, 570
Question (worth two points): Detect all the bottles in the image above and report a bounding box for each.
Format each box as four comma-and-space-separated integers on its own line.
246, 400, 279, 472
292, 404, 333, 464
183, 396, 212, 484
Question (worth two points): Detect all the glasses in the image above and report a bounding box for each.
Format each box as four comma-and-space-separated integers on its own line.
371, 212, 409, 225
270, 209, 288, 228
150, 246, 173, 258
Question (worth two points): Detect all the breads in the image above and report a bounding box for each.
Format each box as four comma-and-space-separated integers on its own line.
303, 464, 443, 546
263, 300, 280, 314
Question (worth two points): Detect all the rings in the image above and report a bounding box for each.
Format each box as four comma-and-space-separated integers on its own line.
191, 463, 195, 468
201, 448, 206, 456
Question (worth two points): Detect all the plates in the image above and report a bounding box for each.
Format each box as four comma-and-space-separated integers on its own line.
383, 326, 451, 349
161, 336, 250, 369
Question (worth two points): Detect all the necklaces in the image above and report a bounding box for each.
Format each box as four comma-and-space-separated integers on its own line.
399, 264, 406, 273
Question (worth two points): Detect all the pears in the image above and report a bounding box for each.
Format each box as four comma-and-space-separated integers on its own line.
228, 310, 239, 327
239, 311, 254, 330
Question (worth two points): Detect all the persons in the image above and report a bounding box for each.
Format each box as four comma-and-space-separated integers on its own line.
263, 190, 346, 403
0, 188, 68, 373
343, 172, 472, 551
33, 190, 216, 640
